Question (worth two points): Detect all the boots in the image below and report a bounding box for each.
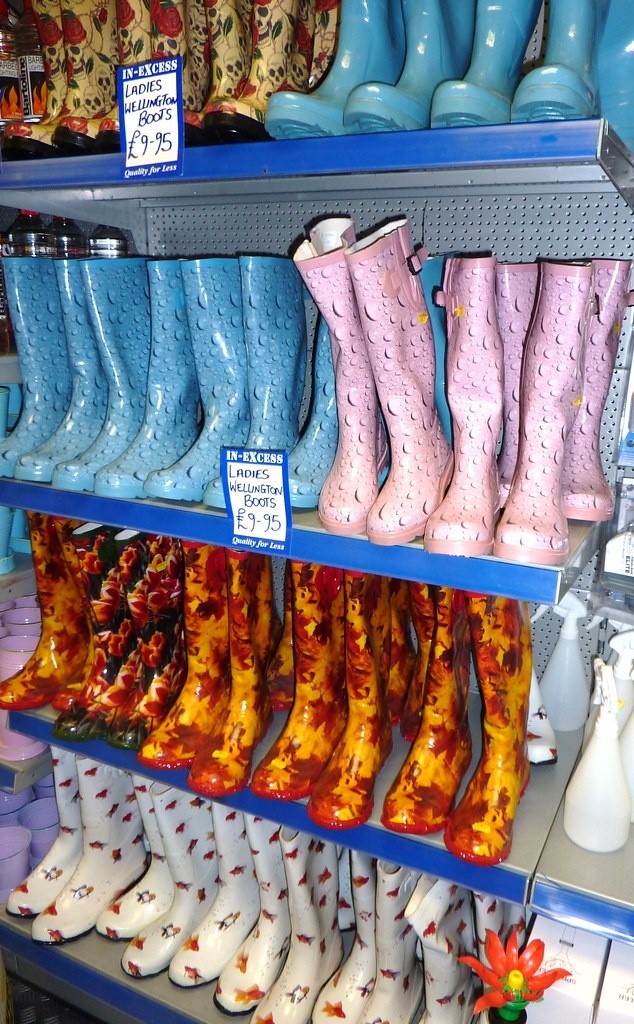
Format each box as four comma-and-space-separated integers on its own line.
0, 2, 634, 1024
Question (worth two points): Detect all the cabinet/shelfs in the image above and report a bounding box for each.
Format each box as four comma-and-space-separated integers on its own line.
0, 117, 634, 1024
